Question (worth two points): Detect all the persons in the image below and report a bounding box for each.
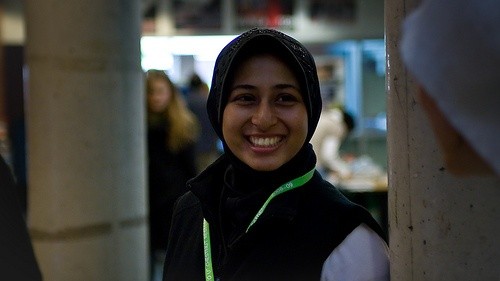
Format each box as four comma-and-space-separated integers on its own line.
308, 107, 355, 182
144, 69, 202, 281
185, 75, 218, 162
163, 27, 392, 281
315, 63, 340, 110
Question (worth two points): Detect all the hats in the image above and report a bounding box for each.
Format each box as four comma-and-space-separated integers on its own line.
205, 29, 322, 143
